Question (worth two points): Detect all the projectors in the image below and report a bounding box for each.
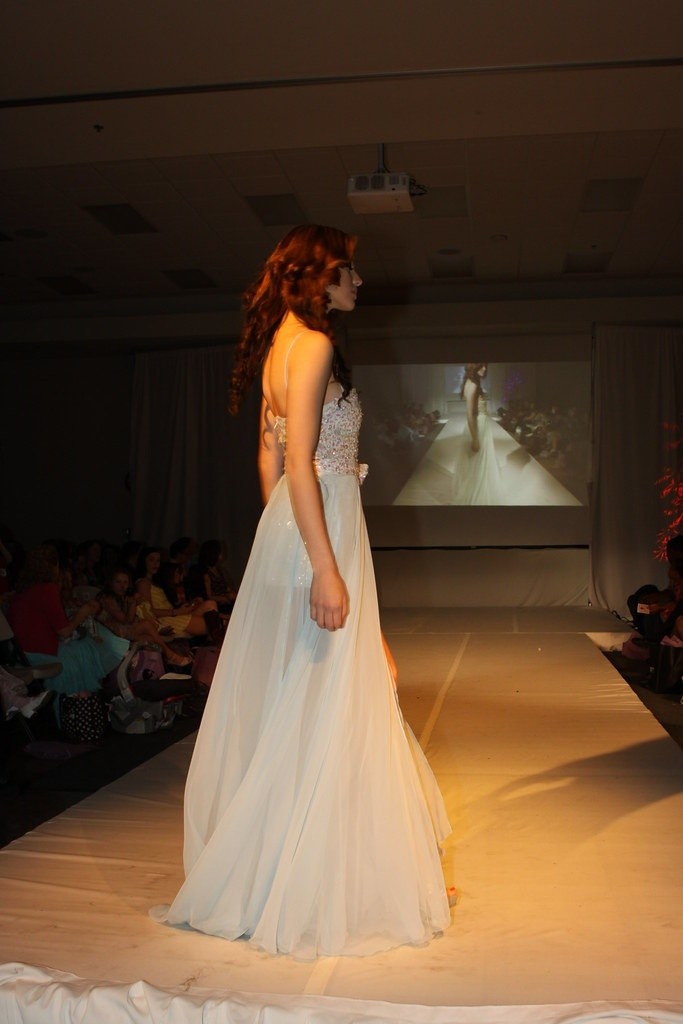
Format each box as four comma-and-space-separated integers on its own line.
346, 173, 417, 214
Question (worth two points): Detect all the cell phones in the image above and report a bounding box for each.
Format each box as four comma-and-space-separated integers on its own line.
637, 603, 649, 613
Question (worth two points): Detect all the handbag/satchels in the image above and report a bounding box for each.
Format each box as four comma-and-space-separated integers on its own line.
654, 644, 683, 692
59, 691, 104, 744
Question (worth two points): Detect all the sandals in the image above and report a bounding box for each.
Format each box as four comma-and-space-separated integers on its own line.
165, 653, 192, 666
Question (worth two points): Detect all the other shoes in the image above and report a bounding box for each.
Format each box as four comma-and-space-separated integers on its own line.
632, 637, 648, 647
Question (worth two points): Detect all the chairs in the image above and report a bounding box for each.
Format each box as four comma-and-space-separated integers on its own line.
0, 608, 62, 742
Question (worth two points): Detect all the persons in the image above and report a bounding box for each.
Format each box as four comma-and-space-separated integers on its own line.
452, 362, 501, 506
494, 397, 576, 456
374, 397, 441, 450
147, 222, 459, 959
0, 522, 236, 742
626, 532, 683, 696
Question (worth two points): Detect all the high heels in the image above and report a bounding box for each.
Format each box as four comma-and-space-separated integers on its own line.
20, 689, 49, 721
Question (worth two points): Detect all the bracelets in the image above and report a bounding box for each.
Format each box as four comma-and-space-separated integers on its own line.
171, 608, 175, 616
